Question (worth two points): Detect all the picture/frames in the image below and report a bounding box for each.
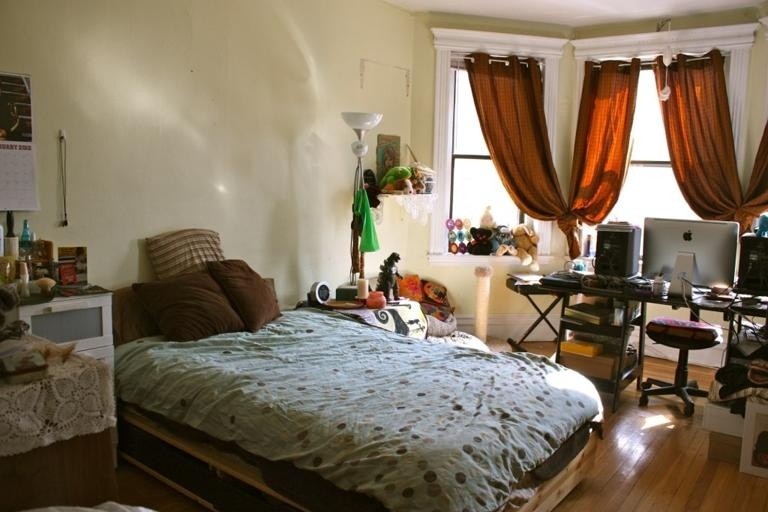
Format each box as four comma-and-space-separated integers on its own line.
738, 401, 768, 479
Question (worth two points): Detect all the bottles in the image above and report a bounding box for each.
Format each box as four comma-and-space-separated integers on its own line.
653, 273, 666, 298
21, 219, 32, 241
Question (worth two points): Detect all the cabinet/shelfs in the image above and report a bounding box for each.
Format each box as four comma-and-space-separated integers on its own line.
1, 333, 113, 512
18, 288, 114, 388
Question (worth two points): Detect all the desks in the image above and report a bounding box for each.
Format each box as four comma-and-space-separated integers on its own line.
540, 270, 768, 413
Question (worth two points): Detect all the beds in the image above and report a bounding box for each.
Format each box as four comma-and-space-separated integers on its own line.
112, 306, 603, 512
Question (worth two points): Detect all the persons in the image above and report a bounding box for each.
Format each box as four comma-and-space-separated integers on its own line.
383, 144, 396, 171
752, 431, 768, 469
1, 100, 22, 141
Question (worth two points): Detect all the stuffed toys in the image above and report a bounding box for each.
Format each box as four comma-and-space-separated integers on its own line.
380, 166, 412, 189
468, 205, 540, 272
412, 181, 424, 191
398, 179, 416, 194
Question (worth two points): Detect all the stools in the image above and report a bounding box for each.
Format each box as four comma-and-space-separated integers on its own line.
639, 315, 724, 417
505, 275, 561, 352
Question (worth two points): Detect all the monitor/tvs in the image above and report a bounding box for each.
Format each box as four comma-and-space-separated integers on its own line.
642, 218, 740, 301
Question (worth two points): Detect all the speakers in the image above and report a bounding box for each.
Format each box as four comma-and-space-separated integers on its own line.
594, 221, 642, 278
732, 233, 768, 296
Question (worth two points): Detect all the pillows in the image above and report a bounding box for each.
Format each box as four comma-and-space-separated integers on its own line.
131, 273, 246, 342
205, 259, 284, 333
145, 228, 225, 281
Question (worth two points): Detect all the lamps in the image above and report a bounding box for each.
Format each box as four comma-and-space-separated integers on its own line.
336, 111, 384, 301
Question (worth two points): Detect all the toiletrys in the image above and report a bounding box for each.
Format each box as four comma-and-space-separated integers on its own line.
0, 219, 57, 298
